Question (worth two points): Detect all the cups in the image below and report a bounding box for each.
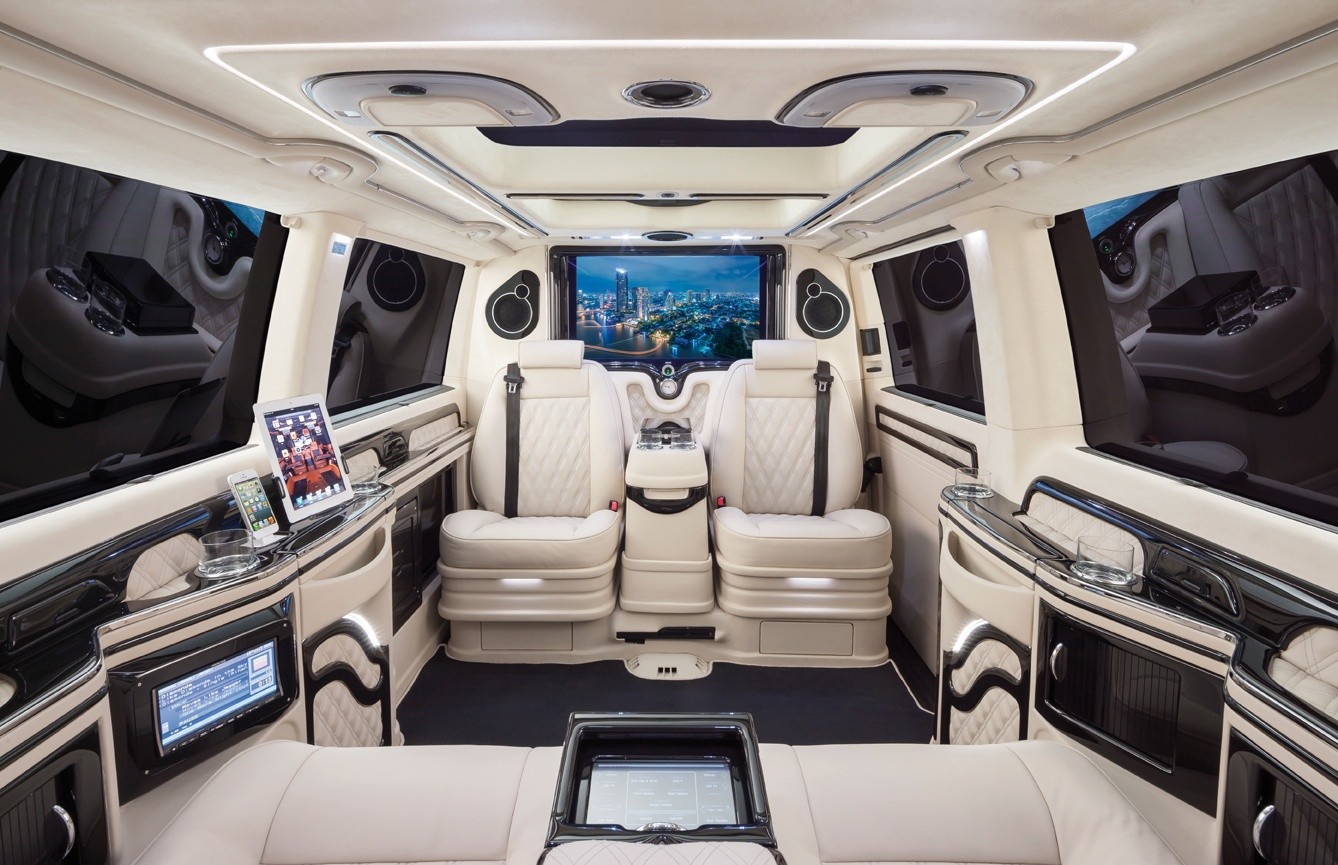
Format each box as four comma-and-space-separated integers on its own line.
639, 428, 662, 452
346, 462, 380, 495
670, 428, 696, 451
197, 528, 255, 576
954, 466, 992, 496
1074, 535, 1135, 585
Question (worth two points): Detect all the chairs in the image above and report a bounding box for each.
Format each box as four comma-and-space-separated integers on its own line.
706, 337, 893, 617
1176, 156, 1338, 344
436, 341, 625, 623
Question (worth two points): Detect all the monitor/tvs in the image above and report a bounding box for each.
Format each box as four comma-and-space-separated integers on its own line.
1082, 186, 1173, 241
218, 199, 266, 247
569, 255, 767, 360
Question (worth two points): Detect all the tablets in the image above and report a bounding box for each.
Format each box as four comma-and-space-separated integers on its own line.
252, 392, 355, 523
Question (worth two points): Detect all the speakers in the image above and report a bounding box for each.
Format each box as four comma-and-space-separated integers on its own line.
921, 244, 965, 305
805, 283, 843, 332
647, 234, 688, 241
373, 246, 417, 305
640, 85, 695, 101
493, 284, 532, 331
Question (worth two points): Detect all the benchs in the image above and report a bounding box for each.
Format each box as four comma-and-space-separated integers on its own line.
124, 738, 1184, 865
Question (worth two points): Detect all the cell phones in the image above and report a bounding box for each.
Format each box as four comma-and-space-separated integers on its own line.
226, 468, 280, 540
